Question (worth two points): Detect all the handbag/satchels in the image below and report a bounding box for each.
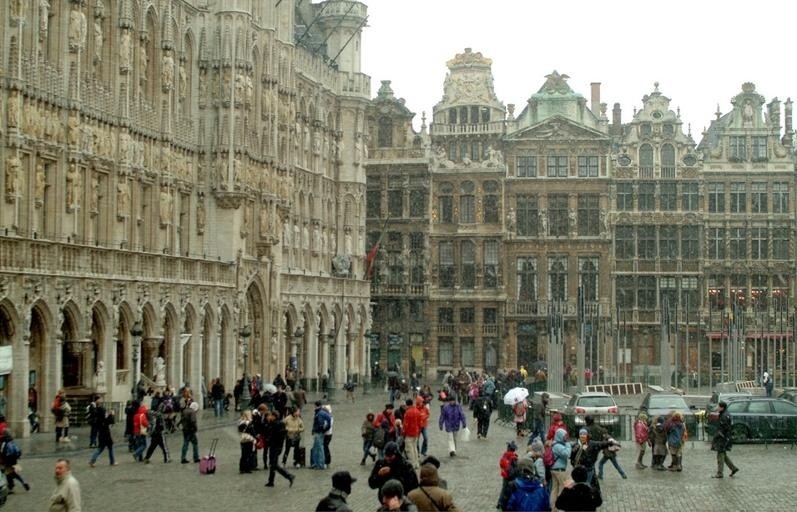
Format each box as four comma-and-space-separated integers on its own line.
241, 433, 254, 443
292, 436, 301, 446
461, 428, 470, 441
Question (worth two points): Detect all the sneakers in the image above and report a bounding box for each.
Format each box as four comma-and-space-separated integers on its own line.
179, 458, 201, 463
88, 460, 118, 467
636, 461, 683, 472
266, 483, 275, 487
360, 453, 376, 465
24, 482, 30, 490
712, 468, 738, 478
59, 436, 71, 442
289, 475, 296, 487
132, 452, 149, 464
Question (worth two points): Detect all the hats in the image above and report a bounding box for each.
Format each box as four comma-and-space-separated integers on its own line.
332, 471, 356, 487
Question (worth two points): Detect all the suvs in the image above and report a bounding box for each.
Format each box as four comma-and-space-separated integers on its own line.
564, 391, 623, 437
702, 389, 797, 444
637, 392, 699, 440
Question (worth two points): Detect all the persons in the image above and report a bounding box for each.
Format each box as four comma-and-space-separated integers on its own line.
504, 205, 611, 242
1, 1, 364, 261
763, 372, 774, 398
740, 99, 754, 128
0, 363, 739, 511
434, 144, 505, 169
374, 243, 437, 284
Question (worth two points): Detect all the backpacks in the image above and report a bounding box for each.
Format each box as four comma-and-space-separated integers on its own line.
544, 443, 565, 466
478, 399, 490, 415
513, 401, 525, 417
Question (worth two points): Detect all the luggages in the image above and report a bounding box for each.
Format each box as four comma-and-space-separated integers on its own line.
300, 447, 306, 466
200, 439, 219, 474
